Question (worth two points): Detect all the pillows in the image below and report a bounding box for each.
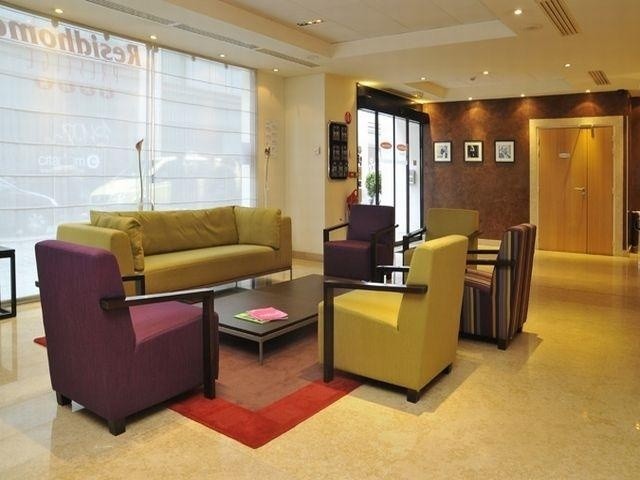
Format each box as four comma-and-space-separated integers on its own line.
234, 206, 281, 249
91, 214, 145, 271
87, 206, 239, 255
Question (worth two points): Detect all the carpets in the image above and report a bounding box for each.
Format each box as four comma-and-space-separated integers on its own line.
34, 322, 364, 449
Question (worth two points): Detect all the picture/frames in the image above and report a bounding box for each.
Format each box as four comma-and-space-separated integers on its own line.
463, 139, 484, 163
494, 139, 516, 163
433, 140, 453, 163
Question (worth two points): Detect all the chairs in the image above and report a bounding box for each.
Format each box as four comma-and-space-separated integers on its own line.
459, 223, 537, 350
35, 238, 220, 435
323, 204, 399, 282
402, 208, 480, 270
318, 234, 468, 402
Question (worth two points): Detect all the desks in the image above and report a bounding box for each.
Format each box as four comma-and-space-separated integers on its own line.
1, 247, 17, 319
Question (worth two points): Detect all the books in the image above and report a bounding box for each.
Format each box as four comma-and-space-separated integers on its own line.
235, 306, 289, 325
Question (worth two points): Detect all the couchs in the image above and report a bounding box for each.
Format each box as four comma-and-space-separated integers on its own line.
57, 205, 293, 294
